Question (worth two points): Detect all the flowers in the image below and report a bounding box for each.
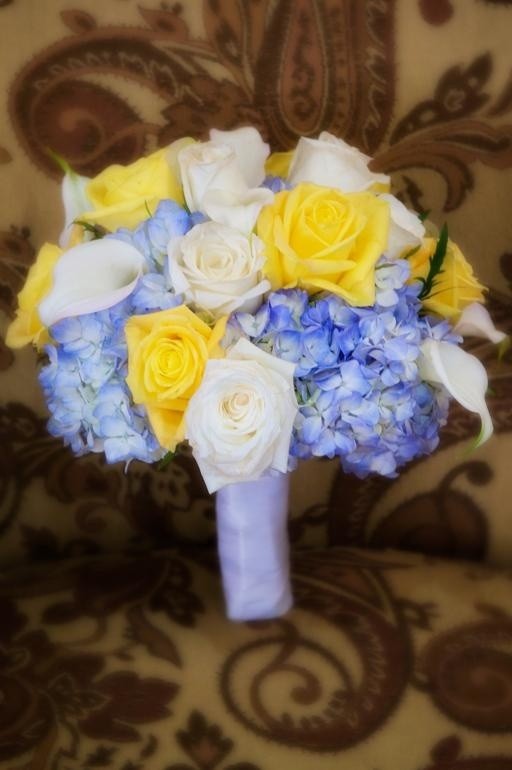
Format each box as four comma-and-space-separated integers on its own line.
5, 123, 510, 622
